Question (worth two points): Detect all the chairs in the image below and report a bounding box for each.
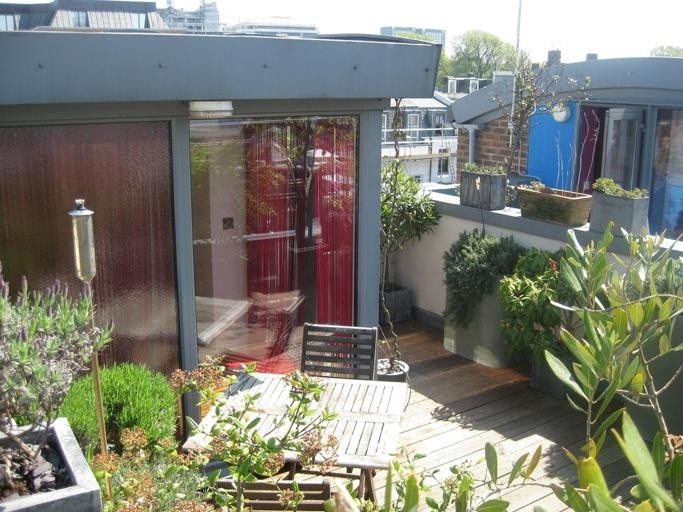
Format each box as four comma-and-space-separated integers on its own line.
298, 320, 377, 379
217, 295, 310, 372
209, 476, 332, 510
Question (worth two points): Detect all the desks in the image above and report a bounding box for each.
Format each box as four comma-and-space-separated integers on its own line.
106, 290, 251, 369
182, 368, 407, 510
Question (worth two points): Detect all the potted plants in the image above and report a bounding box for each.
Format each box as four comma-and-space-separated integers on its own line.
0, 272, 107, 510
